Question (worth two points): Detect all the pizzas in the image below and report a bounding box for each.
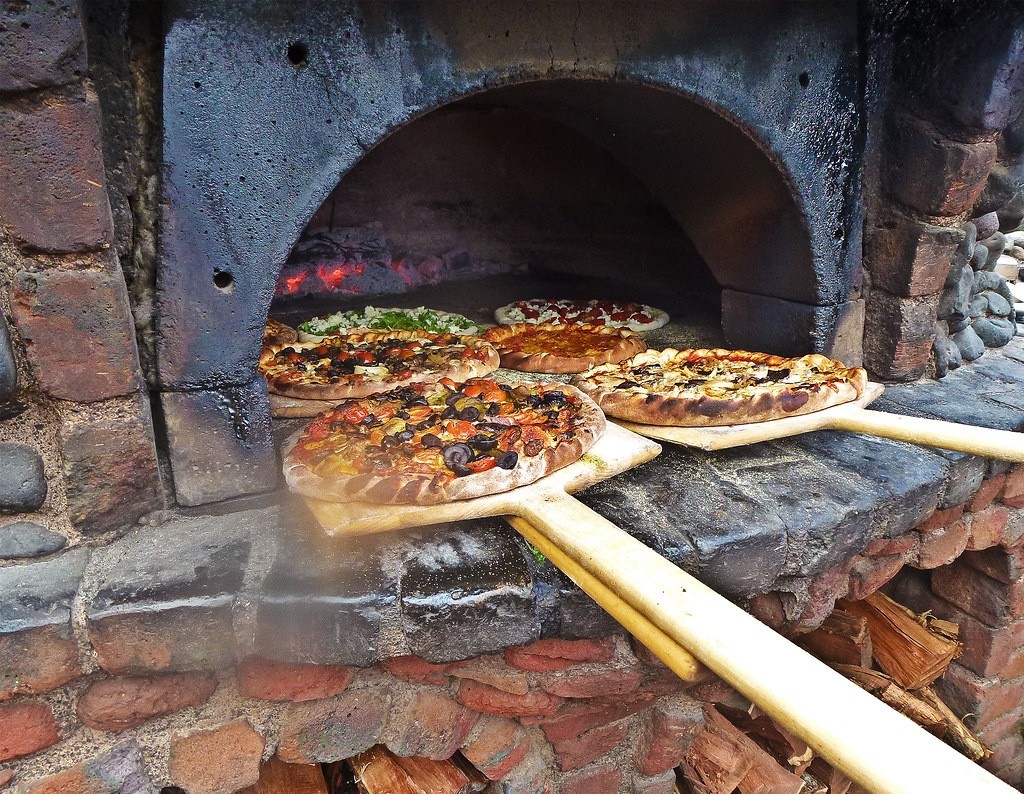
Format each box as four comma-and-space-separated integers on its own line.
258, 318, 297, 369
477, 322, 647, 374
496, 298, 669, 330
257, 330, 500, 398
568, 346, 868, 427
282, 376, 607, 505
296, 306, 479, 343
